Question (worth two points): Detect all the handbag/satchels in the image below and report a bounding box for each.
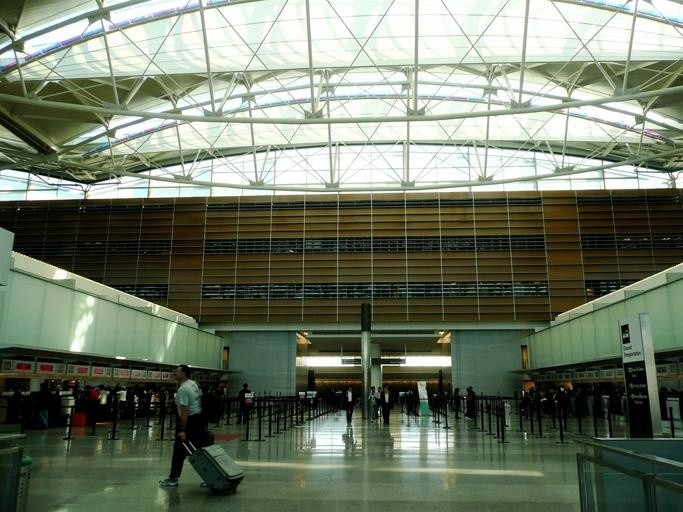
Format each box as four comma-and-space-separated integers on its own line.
193, 431, 214, 448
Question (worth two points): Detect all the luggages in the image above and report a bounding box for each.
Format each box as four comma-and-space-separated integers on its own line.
178, 435, 246, 495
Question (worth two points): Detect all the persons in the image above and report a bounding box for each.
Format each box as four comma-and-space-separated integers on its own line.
158, 364, 213, 486
344, 386, 355, 423
235, 383, 251, 424
520, 384, 626, 420
659, 386, 683, 420
37, 383, 229, 430
369, 385, 392, 425
452, 384, 475, 421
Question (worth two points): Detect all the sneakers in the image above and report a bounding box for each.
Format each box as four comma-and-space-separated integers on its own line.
160, 479, 178, 487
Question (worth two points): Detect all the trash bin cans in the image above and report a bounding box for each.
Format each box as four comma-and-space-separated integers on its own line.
0, 434, 32, 511
